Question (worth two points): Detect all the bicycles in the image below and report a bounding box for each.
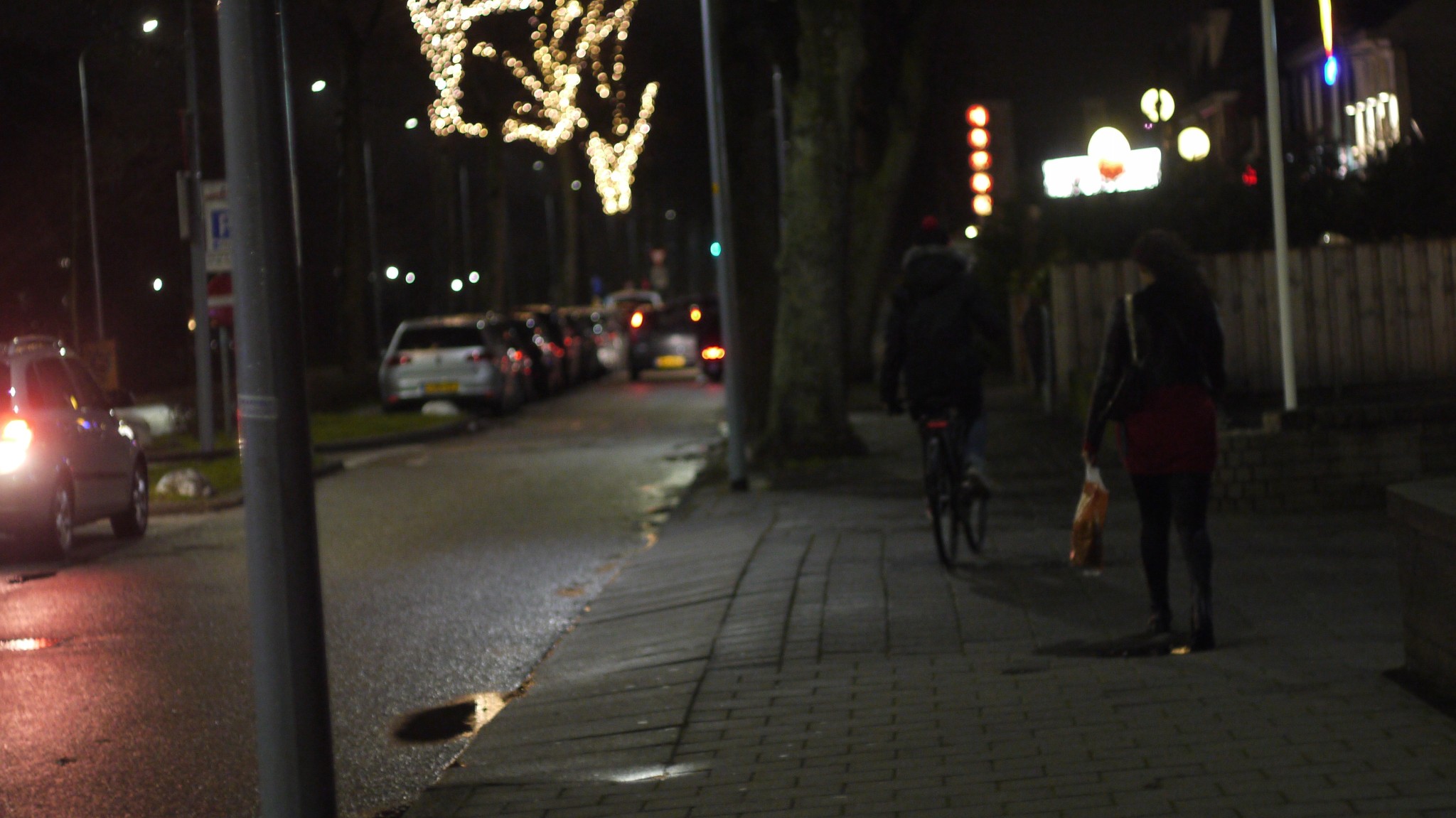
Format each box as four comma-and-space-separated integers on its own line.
916, 409, 990, 569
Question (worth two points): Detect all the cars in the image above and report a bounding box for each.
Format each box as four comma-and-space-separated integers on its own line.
376, 318, 502, 420
477, 285, 665, 408
0, 335, 150, 560
627, 301, 723, 381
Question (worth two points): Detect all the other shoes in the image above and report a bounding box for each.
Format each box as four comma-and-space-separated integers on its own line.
1136, 618, 1171, 653
968, 466, 994, 498
921, 475, 946, 523
1190, 615, 1214, 651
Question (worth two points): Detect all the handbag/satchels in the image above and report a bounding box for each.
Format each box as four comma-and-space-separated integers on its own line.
1107, 362, 1150, 421
1068, 464, 1110, 570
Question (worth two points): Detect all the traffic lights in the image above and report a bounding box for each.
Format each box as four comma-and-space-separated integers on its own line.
382, 263, 481, 293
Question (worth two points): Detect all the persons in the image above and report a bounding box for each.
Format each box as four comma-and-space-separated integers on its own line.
1079, 232, 1227, 650
884, 217, 1012, 523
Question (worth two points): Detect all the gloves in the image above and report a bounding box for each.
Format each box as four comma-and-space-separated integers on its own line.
883, 387, 905, 414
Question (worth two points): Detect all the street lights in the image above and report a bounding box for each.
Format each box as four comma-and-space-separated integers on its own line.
74, 12, 166, 343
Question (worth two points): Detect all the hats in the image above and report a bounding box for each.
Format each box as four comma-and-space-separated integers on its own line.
917, 215, 947, 246
